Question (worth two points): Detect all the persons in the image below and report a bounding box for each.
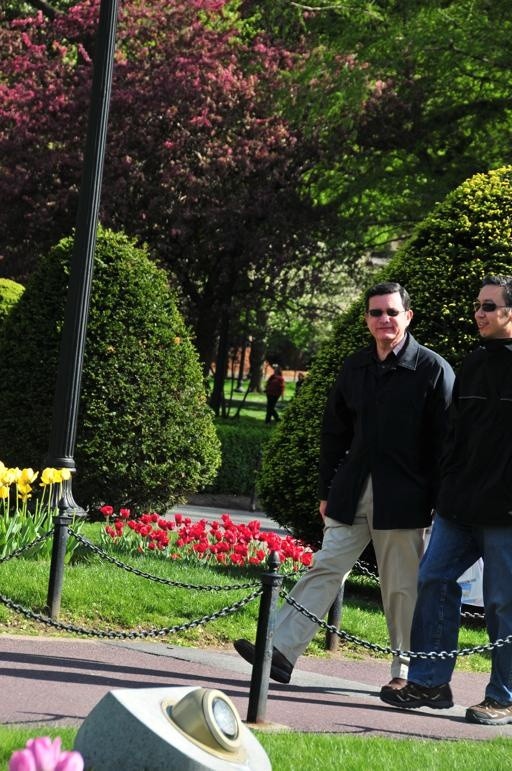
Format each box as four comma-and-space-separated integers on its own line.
295, 372, 304, 393
233, 284, 458, 698
380, 273, 512, 726
262, 367, 284, 422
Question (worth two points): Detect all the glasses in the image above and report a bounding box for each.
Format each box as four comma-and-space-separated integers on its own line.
366, 308, 403, 317
472, 302, 506, 312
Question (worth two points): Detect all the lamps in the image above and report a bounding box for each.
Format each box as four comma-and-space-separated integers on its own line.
161, 685, 249, 763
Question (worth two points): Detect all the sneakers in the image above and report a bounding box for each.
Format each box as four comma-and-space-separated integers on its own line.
236, 638, 294, 683
381, 678, 511, 724
